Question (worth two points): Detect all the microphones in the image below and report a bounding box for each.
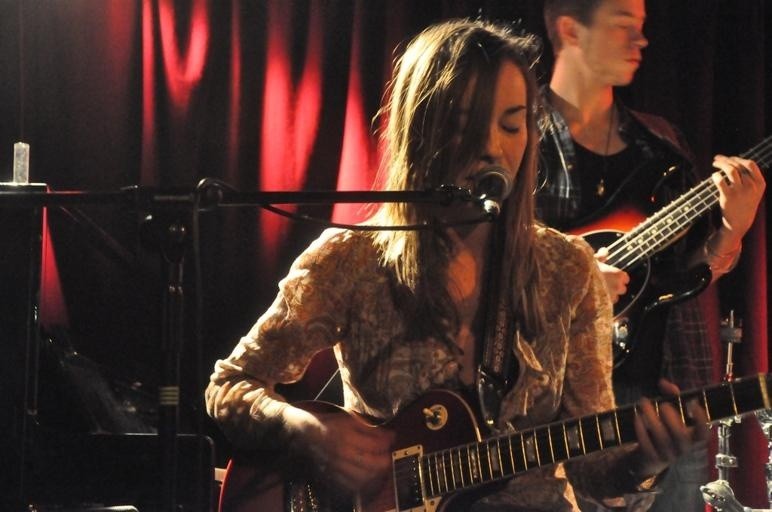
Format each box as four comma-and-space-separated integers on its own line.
474, 164, 513, 215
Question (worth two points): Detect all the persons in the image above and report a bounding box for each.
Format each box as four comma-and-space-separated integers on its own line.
531, 1, 769, 512
203, 17, 711, 511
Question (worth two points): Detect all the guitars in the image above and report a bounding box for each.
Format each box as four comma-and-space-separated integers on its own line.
558, 137, 771, 372
219, 373, 772, 511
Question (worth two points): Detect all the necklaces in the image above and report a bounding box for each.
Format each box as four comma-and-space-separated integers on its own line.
594, 102, 616, 197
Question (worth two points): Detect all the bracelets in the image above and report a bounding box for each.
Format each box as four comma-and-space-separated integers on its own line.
704, 243, 742, 273
702, 231, 741, 258
